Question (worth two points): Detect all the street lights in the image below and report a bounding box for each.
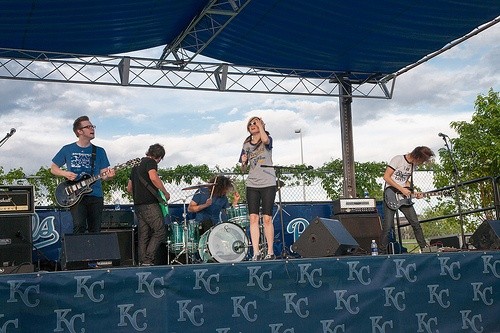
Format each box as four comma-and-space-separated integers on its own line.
295, 129, 307, 201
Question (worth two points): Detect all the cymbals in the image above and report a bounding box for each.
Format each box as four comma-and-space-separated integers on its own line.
275, 181, 284, 192
182, 184, 216, 190
167, 193, 200, 205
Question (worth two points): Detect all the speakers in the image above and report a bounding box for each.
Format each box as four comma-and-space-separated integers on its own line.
469, 219, 500, 250
103, 229, 135, 266
60, 231, 121, 270
0, 213, 33, 266
332, 214, 384, 252
291, 216, 359, 257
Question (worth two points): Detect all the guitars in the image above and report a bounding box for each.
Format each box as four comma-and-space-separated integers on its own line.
53, 158, 142, 208
158, 175, 169, 219
383, 186, 452, 211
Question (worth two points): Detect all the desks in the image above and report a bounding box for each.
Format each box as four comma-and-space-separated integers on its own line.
98, 228, 136, 267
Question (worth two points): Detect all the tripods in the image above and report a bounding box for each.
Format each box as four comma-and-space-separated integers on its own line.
169, 188, 202, 265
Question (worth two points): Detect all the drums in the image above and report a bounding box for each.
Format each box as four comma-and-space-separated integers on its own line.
198, 221, 250, 263
169, 219, 200, 254
225, 203, 250, 228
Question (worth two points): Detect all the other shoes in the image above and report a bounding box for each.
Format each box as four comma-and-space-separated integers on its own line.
264, 254, 275, 260
249, 254, 261, 260
88, 262, 100, 269
142, 262, 155, 266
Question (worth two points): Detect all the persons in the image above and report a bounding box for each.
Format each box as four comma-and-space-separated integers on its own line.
378, 147, 433, 255
49, 115, 116, 269
187, 175, 240, 237
238, 117, 277, 261
127, 144, 170, 266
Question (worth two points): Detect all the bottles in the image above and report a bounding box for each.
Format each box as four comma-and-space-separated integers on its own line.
371, 239, 378, 256
364, 188, 369, 198
114, 197, 120, 210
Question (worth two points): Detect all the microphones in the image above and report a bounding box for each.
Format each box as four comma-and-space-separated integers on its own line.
439, 133, 448, 137
16, 230, 22, 237
243, 150, 249, 166
8, 128, 16, 136
235, 243, 244, 251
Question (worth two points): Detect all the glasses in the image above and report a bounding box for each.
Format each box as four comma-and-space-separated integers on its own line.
79, 126, 96, 130
248, 122, 256, 126
